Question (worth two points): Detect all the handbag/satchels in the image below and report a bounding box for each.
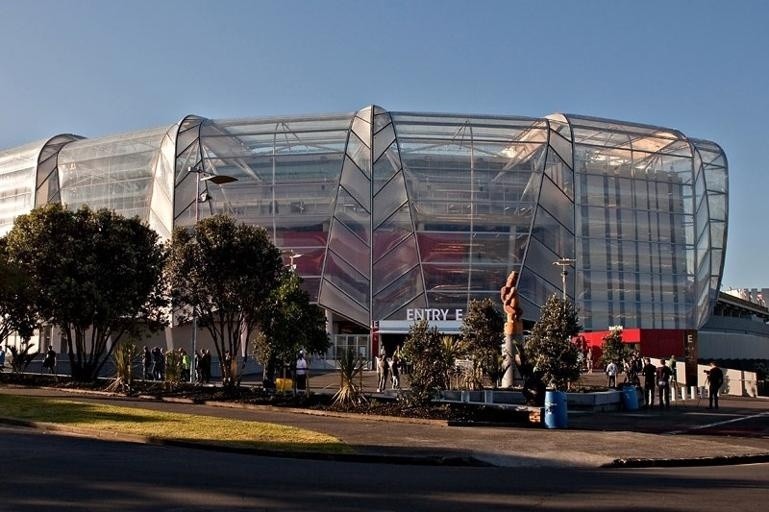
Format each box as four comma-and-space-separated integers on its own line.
659, 381, 665, 387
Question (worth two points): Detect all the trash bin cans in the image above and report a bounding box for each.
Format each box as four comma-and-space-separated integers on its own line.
545, 391, 568, 429
297, 374, 306, 389
623, 387, 640, 411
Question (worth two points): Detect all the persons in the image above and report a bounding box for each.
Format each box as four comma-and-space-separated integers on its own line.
141, 345, 212, 383
293, 349, 308, 391
628, 359, 641, 387
584, 346, 595, 373
575, 348, 585, 373
370, 340, 416, 393
706, 359, 724, 411
42, 344, 57, 374
0, 344, 7, 372
655, 359, 673, 409
623, 361, 630, 384
642, 358, 656, 409
603, 358, 620, 388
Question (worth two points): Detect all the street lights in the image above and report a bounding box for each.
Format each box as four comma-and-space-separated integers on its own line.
551, 256, 578, 317
279, 247, 304, 278
184, 164, 240, 385
